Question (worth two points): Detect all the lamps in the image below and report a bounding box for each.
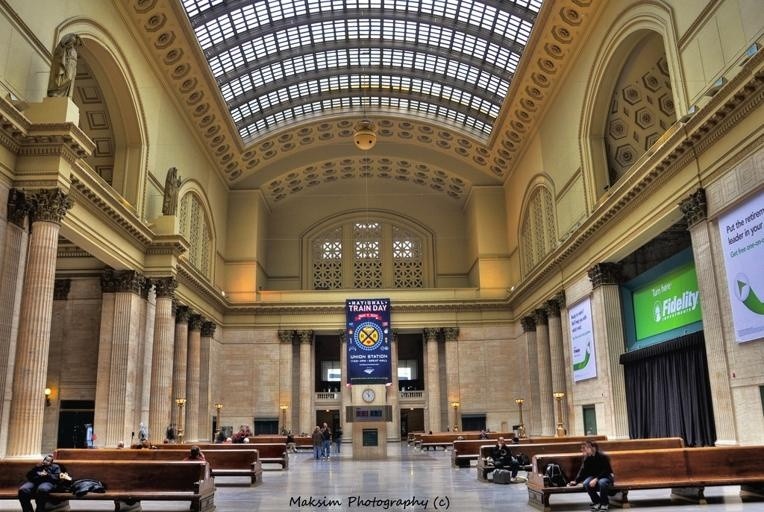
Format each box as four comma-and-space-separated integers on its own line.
353, 66, 377, 150
44, 388, 51, 405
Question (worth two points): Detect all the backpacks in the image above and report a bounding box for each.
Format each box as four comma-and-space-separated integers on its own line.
543, 462, 567, 487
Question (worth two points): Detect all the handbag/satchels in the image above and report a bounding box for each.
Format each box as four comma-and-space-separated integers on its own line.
493, 469, 511, 485
486, 456, 495, 466
515, 453, 531, 465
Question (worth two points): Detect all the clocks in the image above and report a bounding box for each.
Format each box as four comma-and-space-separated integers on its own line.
362, 389, 376, 403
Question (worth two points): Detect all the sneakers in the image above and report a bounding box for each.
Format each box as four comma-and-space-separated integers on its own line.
600, 505, 608, 512
591, 503, 601, 512
511, 478, 517, 482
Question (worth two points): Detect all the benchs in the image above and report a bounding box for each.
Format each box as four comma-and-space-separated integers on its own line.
0, 458, 216, 511
451, 436, 607, 467
526, 445, 764, 512
408, 433, 414, 447
53, 449, 262, 488
420, 432, 516, 451
477, 437, 685, 481
414, 432, 496, 449
248, 437, 314, 449
132, 444, 289, 470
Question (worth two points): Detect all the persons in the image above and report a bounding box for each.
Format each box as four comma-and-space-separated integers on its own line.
218, 424, 252, 443
184, 446, 205, 461
427, 430, 432, 436
479, 429, 489, 439
491, 436, 519, 482
18, 454, 61, 512
72, 423, 93, 449
566, 440, 614, 511
456, 435, 465, 440
511, 437, 519, 444
311, 423, 343, 460
117, 422, 184, 449
286, 435, 296, 453
